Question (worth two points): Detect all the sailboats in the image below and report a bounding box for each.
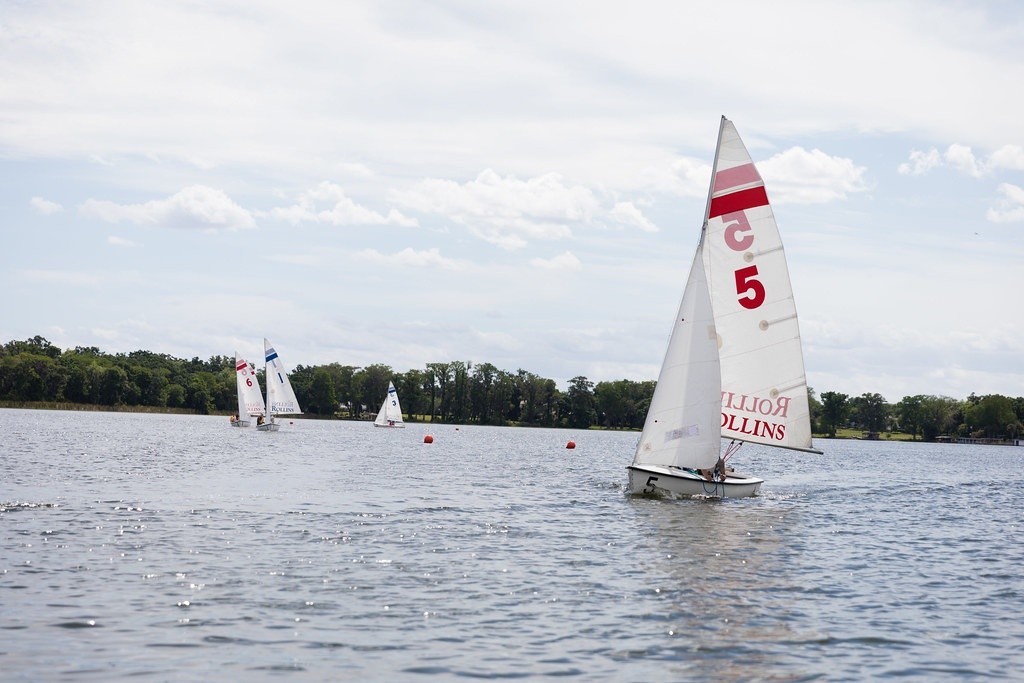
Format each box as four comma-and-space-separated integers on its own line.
254, 337, 305, 431
230, 350, 265, 427
372, 380, 408, 429
622, 113, 826, 499
